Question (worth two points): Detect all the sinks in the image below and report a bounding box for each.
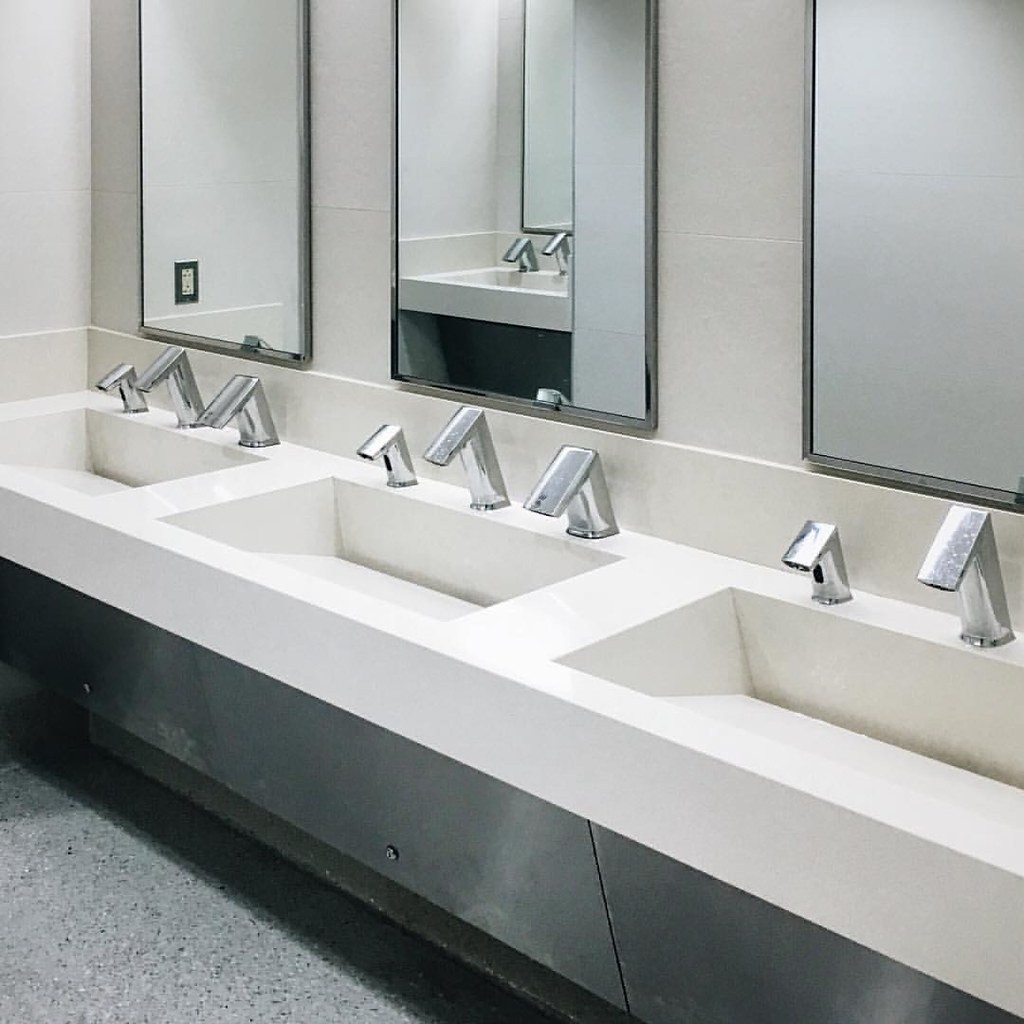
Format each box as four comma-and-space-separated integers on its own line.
431, 268, 570, 297
548, 583, 1024, 838
0, 406, 270, 498
156, 475, 627, 623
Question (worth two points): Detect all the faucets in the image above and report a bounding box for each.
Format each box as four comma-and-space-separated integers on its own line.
421, 405, 513, 512
501, 236, 540, 274
915, 504, 1016, 649
355, 422, 418, 488
540, 232, 571, 276
535, 387, 572, 405
779, 519, 855, 606
93, 361, 149, 413
132, 345, 207, 428
521, 444, 621, 540
193, 373, 281, 448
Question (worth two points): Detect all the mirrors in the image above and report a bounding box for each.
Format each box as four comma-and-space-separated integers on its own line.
520, 0, 575, 236
390, 0, 659, 430
134, 1, 311, 364
799, 1, 1024, 514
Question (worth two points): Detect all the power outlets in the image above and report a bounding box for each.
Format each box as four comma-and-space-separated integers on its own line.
174, 259, 198, 304
